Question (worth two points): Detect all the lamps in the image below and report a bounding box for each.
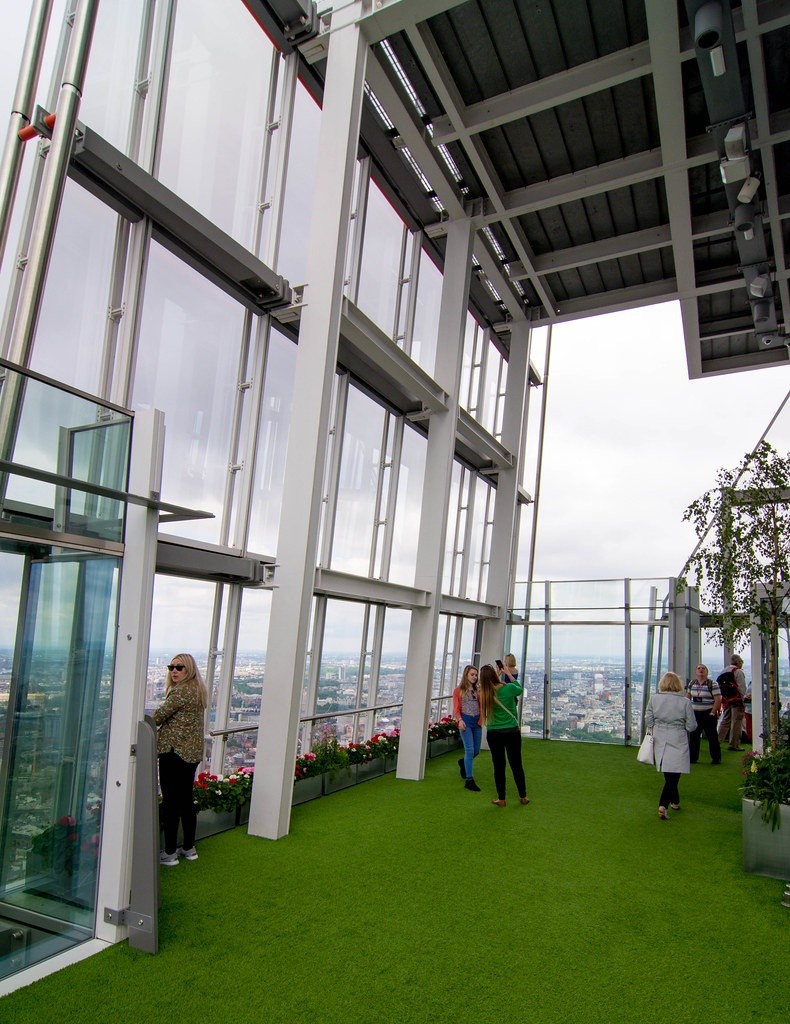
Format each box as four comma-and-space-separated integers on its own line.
753, 301, 770, 323
737, 177, 760, 203
735, 205, 754, 232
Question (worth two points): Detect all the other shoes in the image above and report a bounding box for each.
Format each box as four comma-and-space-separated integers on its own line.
465, 779, 480, 792
691, 759, 697, 763
492, 799, 506, 807
458, 759, 466, 779
711, 759, 720, 765
520, 798, 529, 804
728, 747, 746, 751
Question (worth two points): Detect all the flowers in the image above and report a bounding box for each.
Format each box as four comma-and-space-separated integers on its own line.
314, 716, 348, 768
236, 751, 319, 791
342, 740, 379, 764
428, 714, 459, 738
189, 772, 236, 813
734, 734, 790, 832
36, 805, 99, 877
370, 729, 399, 754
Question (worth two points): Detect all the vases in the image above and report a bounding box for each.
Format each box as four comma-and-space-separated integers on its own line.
356, 758, 386, 783
25, 851, 97, 908
322, 763, 357, 795
239, 793, 251, 826
159, 808, 236, 853
430, 733, 462, 759
384, 743, 431, 773
740, 796, 790, 882
292, 775, 323, 806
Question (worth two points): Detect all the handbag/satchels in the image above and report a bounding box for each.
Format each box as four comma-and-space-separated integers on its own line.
717, 668, 738, 698
637, 726, 654, 765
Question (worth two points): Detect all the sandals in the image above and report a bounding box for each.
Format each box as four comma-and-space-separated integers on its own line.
658, 806, 669, 820
671, 804, 679, 809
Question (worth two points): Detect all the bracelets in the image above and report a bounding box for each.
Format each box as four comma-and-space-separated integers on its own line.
710, 713, 714, 716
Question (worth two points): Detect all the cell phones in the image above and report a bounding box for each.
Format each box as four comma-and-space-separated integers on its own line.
496, 660, 503, 670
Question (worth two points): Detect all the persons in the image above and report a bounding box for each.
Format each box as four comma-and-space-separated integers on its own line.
480, 663, 530, 807
716, 654, 752, 751
151, 654, 208, 866
685, 664, 722, 765
504, 653, 518, 683
643, 672, 698, 819
453, 665, 483, 792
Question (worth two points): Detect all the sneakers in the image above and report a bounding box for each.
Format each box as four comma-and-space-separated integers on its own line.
176, 846, 198, 860
160, 849, 179, 866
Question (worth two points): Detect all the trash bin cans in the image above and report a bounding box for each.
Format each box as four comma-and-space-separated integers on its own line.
742, 694, 753, 737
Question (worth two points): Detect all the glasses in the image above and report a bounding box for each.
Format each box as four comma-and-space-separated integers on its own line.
471, 690, 476, 699
698, 667, 705, 669
167, 665, 185, 671
